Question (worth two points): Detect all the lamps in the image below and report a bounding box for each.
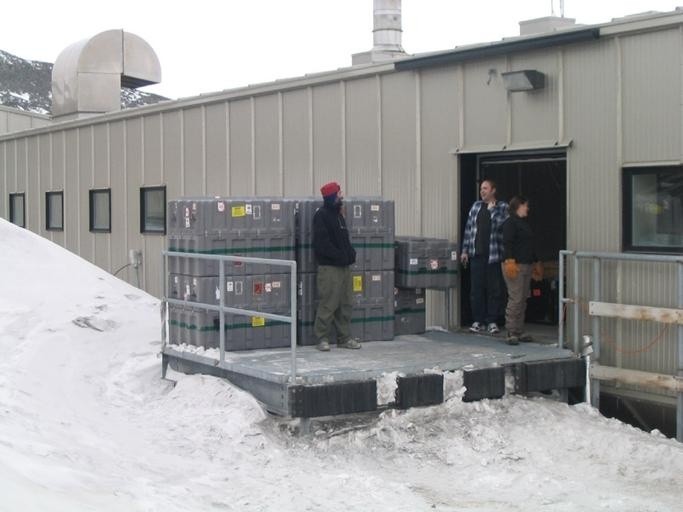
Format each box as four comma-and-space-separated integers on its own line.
497, 69, 547, 92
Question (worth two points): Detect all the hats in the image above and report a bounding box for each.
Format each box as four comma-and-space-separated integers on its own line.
321, 182, 340, 197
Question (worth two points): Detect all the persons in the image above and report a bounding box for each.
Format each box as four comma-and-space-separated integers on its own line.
311, 180, 364, 351
460, 181, 511, 332
496, 194, 546, 344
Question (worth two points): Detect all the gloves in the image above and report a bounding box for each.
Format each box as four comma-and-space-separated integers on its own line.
532, 261, 544, 282
505, 259, 520, 278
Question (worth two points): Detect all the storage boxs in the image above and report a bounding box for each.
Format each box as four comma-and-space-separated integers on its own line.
397, 234, 461, 290
394, 285, 426, 336
298, 272, 394, 345
168, 274, 294, 351
168, 199, 295, 273
297, 197, 395, 270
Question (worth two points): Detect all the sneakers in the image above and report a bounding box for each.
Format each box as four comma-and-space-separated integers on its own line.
337, 339, 360, 349
318, 342, 329, 351
470, 321, 532, 345
